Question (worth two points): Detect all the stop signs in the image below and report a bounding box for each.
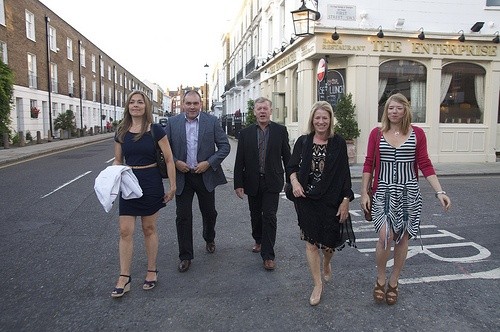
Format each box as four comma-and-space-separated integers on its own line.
316, 58, 327, 82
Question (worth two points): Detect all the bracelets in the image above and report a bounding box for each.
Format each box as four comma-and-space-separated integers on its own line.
435, 191, 447, 199
291, 178, 299, 184
343, 197, 351, 202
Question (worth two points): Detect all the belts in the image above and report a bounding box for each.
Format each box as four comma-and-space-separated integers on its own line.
185, 170, 198, 173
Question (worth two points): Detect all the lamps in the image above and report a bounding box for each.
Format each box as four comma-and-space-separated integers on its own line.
493, 31, 500, 44
331, 26, 340, 40
266, 52, 273, 62
377, 25, 384, 40
272, 48, 280, 57
290, 0, 317, 36
255, 62, 263, 69
470, 22, 484, 33
289, 32, 298, 44
262, 58, 267, 65
456, 30, 467, 42
280, 41, 289, 52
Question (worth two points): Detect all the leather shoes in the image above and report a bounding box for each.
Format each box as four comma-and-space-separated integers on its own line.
264, 259, 274, 270
252, 244, 261, 253
178, 260, 191, 272
206, 241, 215, 253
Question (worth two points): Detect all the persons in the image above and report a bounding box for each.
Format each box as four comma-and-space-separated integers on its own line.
164, 91, 231, 272
359, 94, 451, 306
286, 102, 357, 307
235, 98, 295, 272
110, 91, 179, 299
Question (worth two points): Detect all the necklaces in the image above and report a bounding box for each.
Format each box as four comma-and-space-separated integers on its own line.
389, 128, 403, 135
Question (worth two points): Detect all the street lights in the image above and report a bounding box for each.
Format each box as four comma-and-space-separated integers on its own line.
203, 64, 209, 112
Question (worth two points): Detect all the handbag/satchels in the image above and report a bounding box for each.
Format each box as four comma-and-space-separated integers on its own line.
284, 172, 300, 202
364, 191, 374, 221
155, 146, 168, 178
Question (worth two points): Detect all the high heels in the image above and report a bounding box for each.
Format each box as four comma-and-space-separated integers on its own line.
323, 260, 331, 281
386, 280, 399, 305
310, 283, 322, 305
374, 277, 386, 304
111, 274, 132, 298
142, 268, 159, 290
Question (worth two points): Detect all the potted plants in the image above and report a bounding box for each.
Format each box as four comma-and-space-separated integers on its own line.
112, 120, 123, 132
334, 92, 361, 165
53, 110, 76, 138
